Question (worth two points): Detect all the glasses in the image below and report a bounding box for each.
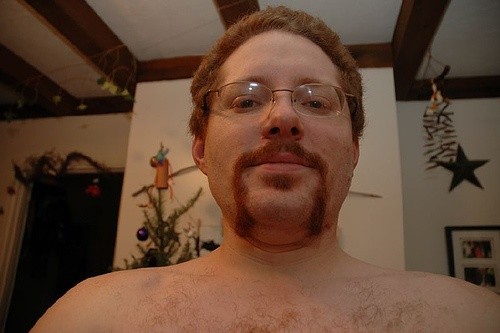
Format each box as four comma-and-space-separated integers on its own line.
201, 81, 357, 117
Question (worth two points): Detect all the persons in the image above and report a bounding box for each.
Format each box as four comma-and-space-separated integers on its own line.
28, 6, 500, 333
461, 237, 496, 287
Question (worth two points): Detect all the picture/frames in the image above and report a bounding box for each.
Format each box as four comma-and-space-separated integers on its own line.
445, 226, 500, 295
